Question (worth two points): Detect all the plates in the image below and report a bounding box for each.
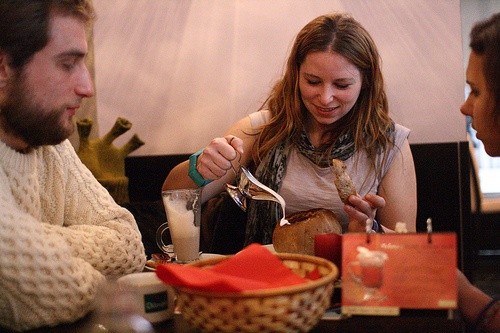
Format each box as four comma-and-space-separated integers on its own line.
145, 253, 225, 271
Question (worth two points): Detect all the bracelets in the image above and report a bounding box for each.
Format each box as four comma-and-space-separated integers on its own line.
372, 218, 385, 234
188, 148, 215, 187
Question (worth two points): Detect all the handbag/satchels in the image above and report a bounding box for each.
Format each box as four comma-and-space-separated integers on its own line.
200, 193, 247, 252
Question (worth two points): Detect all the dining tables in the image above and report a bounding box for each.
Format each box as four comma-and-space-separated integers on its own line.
158, 282, 446, 333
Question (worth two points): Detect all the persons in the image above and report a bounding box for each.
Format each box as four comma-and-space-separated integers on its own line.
456, 17, 500, 333
0, 0, 146, 333
162, 14, 418, 252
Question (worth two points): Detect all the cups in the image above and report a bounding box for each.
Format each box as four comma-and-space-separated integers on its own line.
155, 189, 202, 262
115, 271, 174, 333
346, 251, 387, 302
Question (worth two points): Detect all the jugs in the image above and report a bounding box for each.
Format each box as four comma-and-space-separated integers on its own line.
223, 150, 281, 210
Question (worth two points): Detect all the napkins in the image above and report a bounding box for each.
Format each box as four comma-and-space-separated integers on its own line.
152, 243, 321, 292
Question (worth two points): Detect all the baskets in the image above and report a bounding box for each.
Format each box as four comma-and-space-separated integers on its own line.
166, 252, 338, 333
